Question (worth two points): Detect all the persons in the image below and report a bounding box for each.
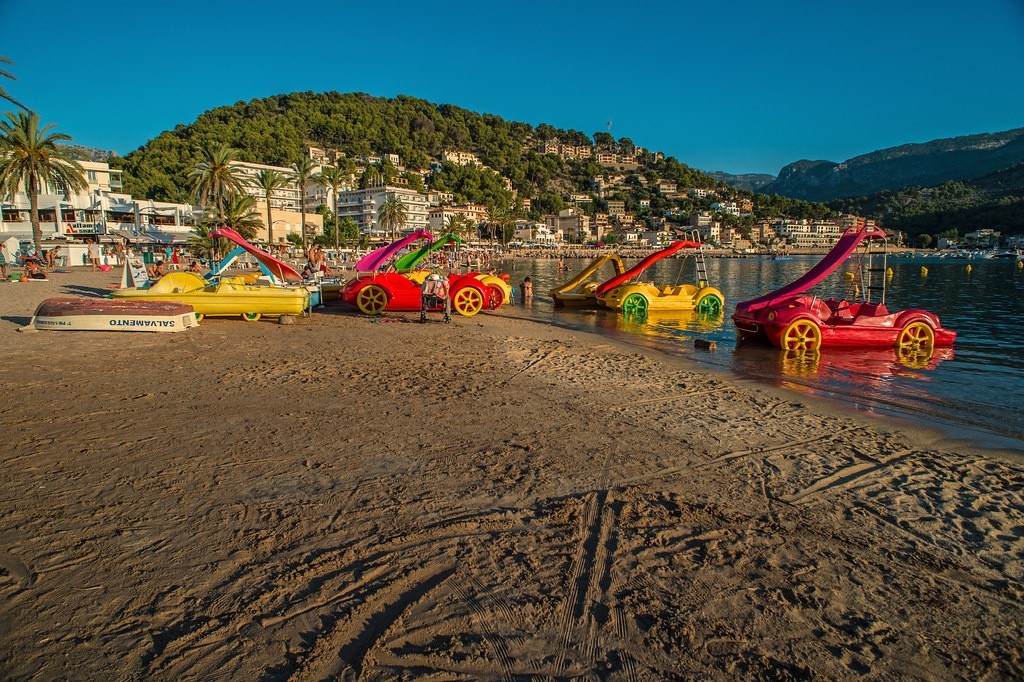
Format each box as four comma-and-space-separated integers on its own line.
519, 277, 533, 297
86, 238, 101, 270
558, 258, 569, 270
255, 241, 381, 280
105, 242, 221, 281
433, 248, 515, 275
45, 246, 61, 272
24, 260, 48, 279
773, 249, 789, 257
0, 241, 9, 280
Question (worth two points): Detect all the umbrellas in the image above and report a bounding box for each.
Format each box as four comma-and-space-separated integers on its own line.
250, 240, 291, 249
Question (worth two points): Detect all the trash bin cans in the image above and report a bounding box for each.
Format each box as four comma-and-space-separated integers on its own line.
144, 252, 154, 263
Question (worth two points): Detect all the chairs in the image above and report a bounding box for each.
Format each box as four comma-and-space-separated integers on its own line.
216, 276, 249, 292
826, 298, 890, 326
659, 285, 687, 295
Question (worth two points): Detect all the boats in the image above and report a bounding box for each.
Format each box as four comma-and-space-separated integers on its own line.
1015, 256, 1024, 270
201, 246, 319, 307
940, 252, 957, 258
972, 251, 994, 259
110, 226, 308, 324
895, 252, 905, 257
904, 251, 915, 259
958, 252, 971, 259
926, 254, 943, 260
17, 297, 200, 332
995, 251, 1016, 257
770, 250, 793, 261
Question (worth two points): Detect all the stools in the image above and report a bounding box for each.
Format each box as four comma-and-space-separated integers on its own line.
298, 264, 306, 270
290, 261, 296, 265
168, 264, 181, 270
337, 266, 346, 272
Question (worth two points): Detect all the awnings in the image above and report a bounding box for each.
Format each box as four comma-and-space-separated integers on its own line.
98, 230, 203, 244
0, 231, 56, 242
